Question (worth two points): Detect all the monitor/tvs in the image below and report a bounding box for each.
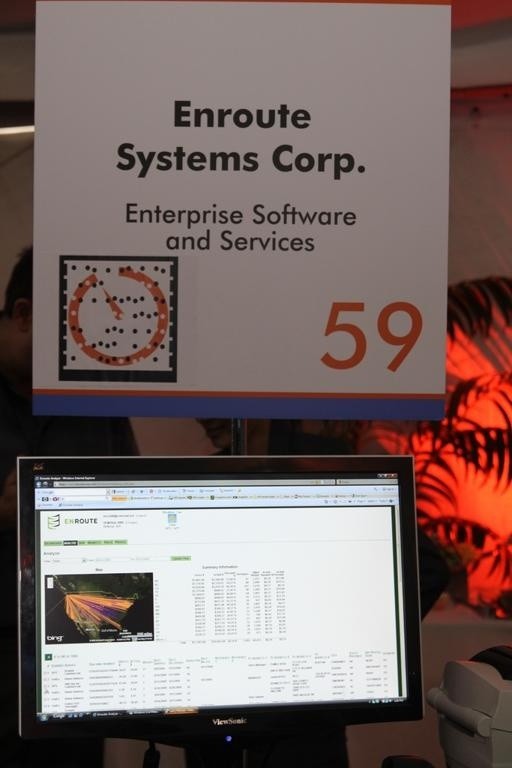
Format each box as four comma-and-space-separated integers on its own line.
16, 452, 424, 768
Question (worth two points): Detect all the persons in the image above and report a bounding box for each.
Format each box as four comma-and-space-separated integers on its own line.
0, 243, 145, 767
169, 415, 453, 768
